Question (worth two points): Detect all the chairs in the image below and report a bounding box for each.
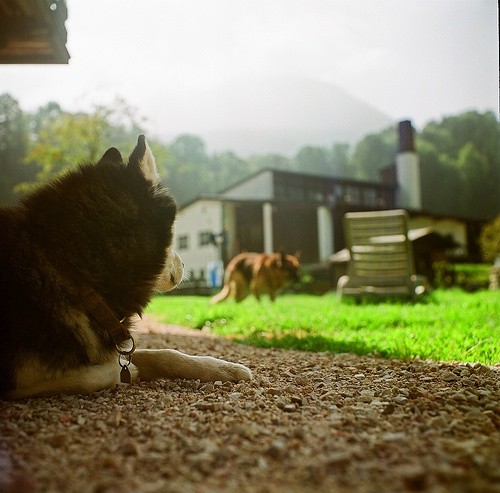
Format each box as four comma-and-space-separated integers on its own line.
335, 205, 418, 307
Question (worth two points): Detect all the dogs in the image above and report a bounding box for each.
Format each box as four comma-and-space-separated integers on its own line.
209, 249, 302, 306
0, 134, 252, 401
